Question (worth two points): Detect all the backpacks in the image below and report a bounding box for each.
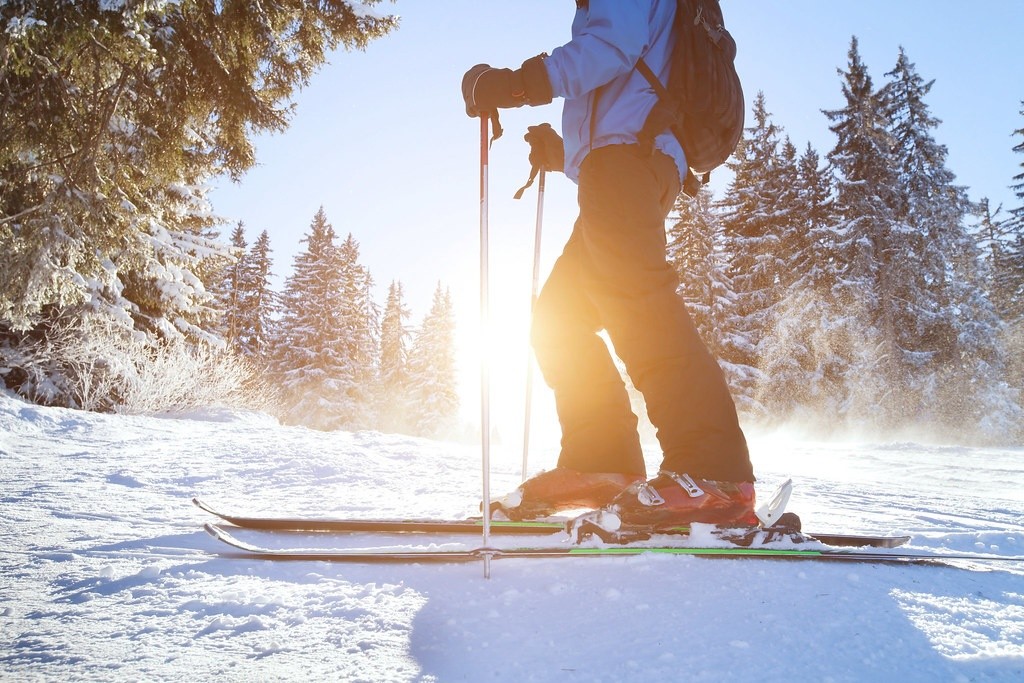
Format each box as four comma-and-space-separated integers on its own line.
585, 0, 744, 172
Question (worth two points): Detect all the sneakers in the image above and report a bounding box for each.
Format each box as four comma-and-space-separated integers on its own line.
609, 472, 760, 531
502, 470, 646, 522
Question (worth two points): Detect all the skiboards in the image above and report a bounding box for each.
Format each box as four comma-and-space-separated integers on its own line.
192, 477, 1024, 562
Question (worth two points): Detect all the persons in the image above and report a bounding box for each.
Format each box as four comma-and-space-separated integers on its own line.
460, 0, 756, 545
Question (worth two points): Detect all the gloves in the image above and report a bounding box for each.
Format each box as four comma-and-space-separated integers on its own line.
462, 63, 525, 118
524, 125, 564, 172
